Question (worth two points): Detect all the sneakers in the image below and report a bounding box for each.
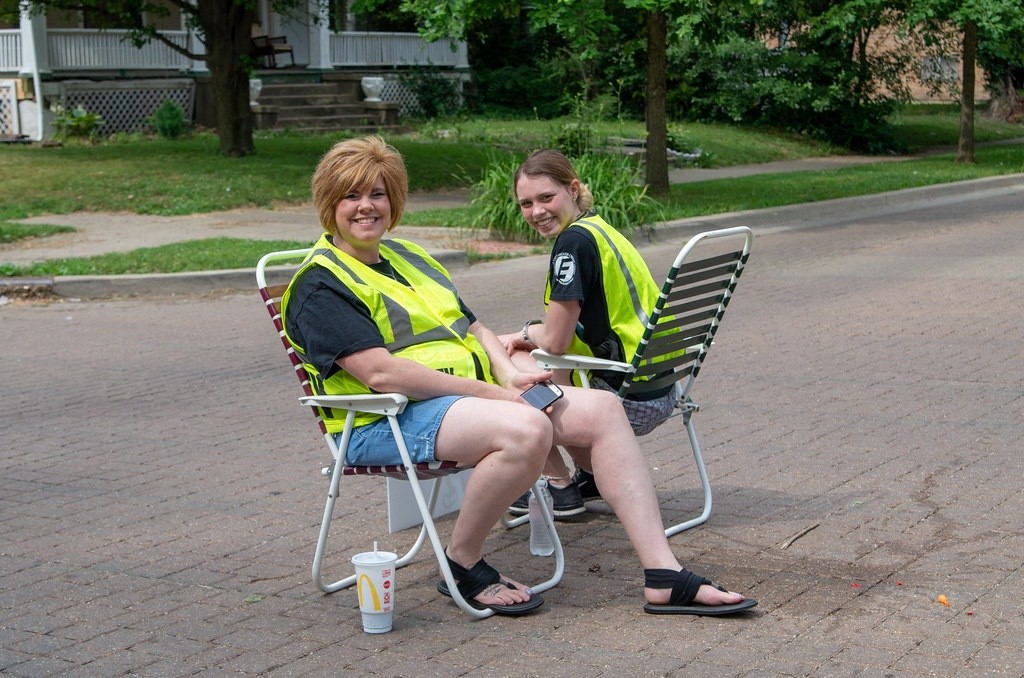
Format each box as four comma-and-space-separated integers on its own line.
508, 469, 586, 516
570, 457, 616, 515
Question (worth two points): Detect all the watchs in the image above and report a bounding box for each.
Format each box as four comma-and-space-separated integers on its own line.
523, 319, 544, 348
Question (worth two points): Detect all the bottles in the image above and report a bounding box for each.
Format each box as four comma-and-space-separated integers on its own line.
528, 480, 555, 556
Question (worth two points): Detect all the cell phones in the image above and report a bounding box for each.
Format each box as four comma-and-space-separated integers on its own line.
520, 379, 563, 411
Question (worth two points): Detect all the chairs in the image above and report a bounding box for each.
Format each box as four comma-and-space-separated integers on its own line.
498, 226, 753, 540
250, 22, 295, 70
255, 249, 564, 619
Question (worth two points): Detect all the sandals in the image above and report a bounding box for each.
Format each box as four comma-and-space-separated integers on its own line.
644, 568, 758, 615
436, 545, 544, 615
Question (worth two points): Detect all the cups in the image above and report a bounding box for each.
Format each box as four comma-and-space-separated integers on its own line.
351, 551, 398, 634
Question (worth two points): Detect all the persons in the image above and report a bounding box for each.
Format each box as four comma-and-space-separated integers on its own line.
279, 137, 758, 615
493, 151, 686, 516
